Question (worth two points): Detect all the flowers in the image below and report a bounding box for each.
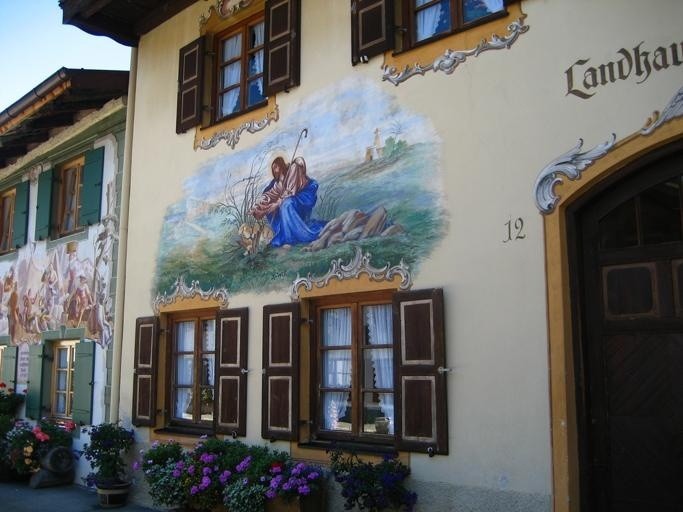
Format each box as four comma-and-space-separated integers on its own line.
135, 433, 321, 502
11, 418, 77, 473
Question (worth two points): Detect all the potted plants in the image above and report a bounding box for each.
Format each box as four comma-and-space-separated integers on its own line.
82, 421, 134, 508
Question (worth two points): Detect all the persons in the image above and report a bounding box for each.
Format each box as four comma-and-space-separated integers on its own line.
251, 157, 329, 249
0, 251, 112, 349
